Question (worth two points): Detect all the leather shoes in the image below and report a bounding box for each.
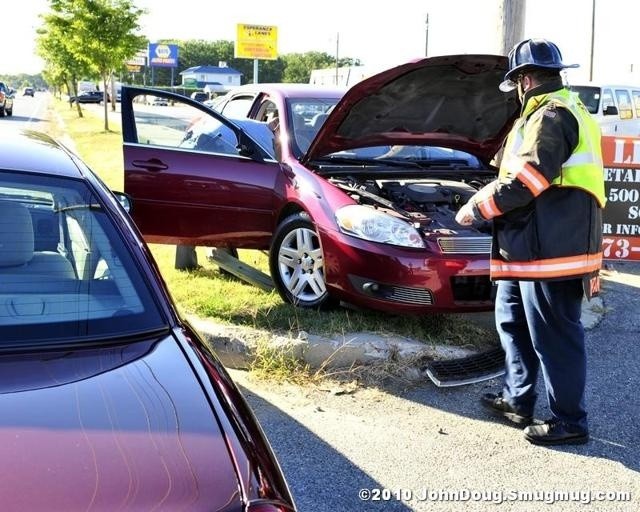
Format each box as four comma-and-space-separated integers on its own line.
480, 392, 537, 423
522, 416, 588, 444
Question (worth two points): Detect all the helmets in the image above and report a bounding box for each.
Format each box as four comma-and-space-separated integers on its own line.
498, 36, 581, 93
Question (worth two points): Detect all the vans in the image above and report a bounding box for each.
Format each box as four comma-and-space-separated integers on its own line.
564, 84, 640, 137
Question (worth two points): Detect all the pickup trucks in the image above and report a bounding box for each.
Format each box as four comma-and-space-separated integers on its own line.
0, 82, 16, 117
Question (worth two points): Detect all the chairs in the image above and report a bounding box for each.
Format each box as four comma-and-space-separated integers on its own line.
0, 200, 77, 280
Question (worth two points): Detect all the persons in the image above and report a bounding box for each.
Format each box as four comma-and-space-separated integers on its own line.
453, 36, 606, 446
174, 110, 295, 275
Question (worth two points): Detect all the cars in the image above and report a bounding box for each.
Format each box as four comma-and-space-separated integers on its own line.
121, 54, 603, 316
23, 88, 35, 97
190, 91, 208, 104
151, 95, 169, 106
68, 86, 121, 105
0, 126, 298, 511
